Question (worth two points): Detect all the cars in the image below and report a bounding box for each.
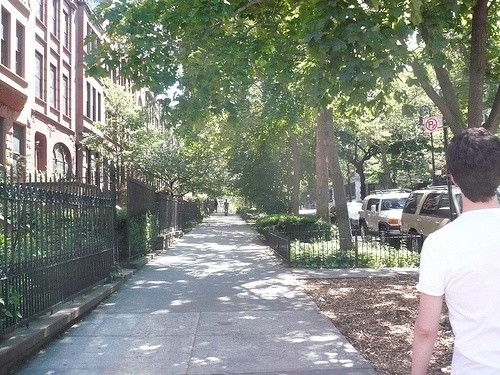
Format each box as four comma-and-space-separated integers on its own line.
329, 201, 362, 235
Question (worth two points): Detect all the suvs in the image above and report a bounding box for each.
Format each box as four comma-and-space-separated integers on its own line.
358, 189, 411, 243
399, 184, 500, 254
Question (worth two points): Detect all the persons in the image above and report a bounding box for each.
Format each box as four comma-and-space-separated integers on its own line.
411, 127, 500, 374
223, 198, 229, 216
